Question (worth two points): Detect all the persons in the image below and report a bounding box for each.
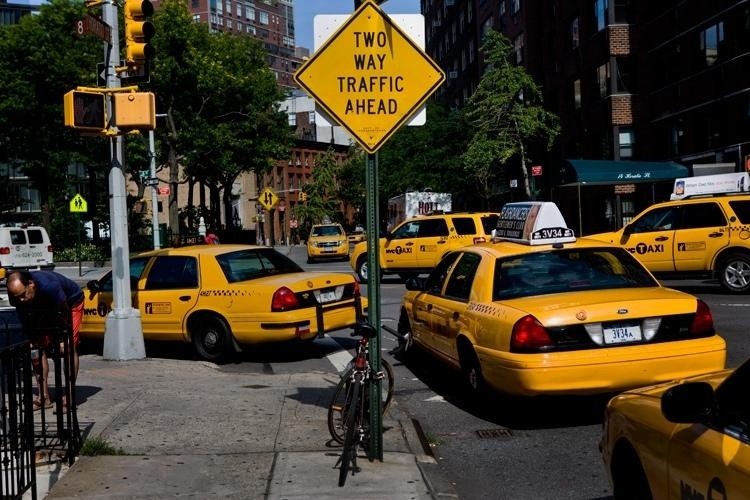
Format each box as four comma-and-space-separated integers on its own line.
659, 223, 671, 230
289, 215, 299, 246
204, 229, 221, 247
380, 218, 393, 234
8, 262, 85, 413
143, 223, 152, 235
604, 198, 612, 232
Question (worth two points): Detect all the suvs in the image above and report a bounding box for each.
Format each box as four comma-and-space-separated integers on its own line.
0, 224, 56, 274
576, 172, 750, 294
351, 210, 501, 284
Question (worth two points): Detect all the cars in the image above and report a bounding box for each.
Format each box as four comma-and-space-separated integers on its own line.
348, 231, 367, 243
398, 202, 728, 396
307, 223, 350, 262
79, 244, 369, 364
599, 356, 749, 499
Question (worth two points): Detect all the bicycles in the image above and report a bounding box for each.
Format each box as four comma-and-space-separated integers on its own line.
329, 324, 394, 487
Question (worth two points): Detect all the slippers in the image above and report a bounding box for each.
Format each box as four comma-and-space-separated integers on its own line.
53, 403, 75, 415
32, 399, 53, 411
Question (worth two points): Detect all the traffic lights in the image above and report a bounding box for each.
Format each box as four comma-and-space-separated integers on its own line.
73, 92, 104, 131
124, 0, 155, 62
